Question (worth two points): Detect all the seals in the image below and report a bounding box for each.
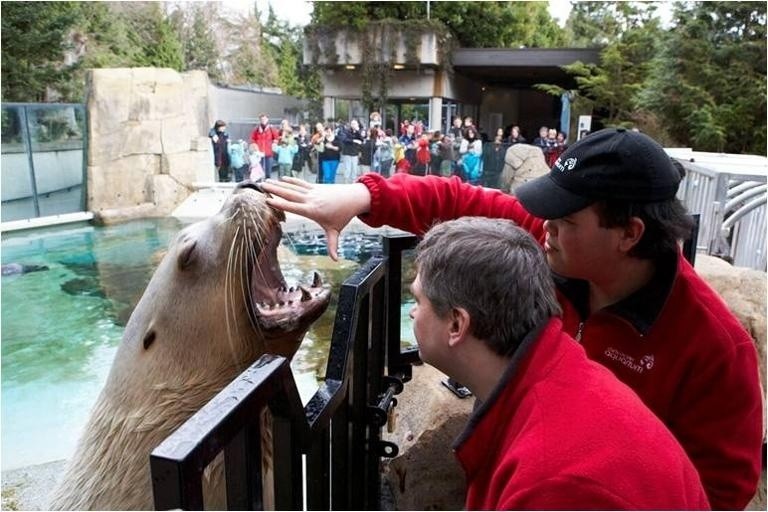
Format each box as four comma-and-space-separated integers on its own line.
39, 182, 333, 511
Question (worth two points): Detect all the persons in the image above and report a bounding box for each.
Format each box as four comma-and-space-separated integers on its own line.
261, 126, 763, 510
408, 215, 711, 510
494, 125, 569, 168
208, 110, 485, 186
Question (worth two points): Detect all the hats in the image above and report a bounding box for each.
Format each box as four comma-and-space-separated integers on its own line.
516, 126, 683, 219
